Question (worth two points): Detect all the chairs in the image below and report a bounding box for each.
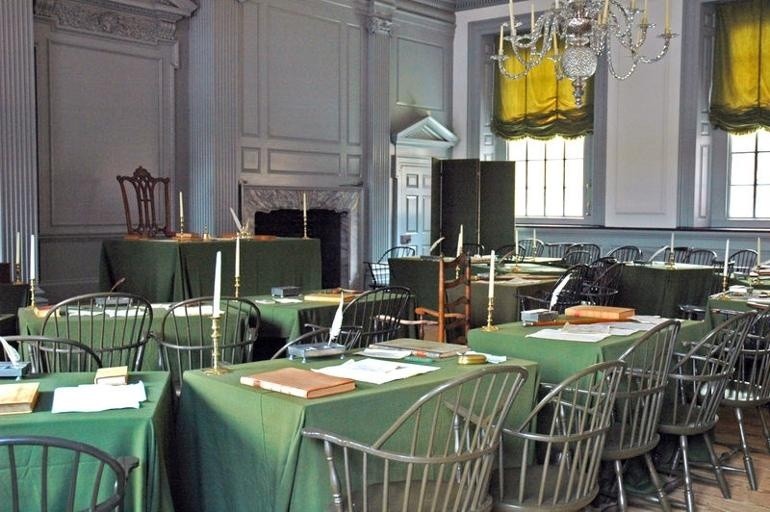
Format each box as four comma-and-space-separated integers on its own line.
114, 164, 172, 234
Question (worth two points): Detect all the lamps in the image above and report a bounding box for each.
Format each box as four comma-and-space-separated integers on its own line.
491, 0, 676, 110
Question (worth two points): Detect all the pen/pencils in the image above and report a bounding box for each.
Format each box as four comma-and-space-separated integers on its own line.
403, 356, 435, 362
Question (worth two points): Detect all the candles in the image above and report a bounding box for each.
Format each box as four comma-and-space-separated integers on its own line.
303, 191, 307, 223
179, 190, 183, 221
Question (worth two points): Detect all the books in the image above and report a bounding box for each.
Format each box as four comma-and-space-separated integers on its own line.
33, 304, 61, 319
0, 381, 42, 417
239, 365, 358, 399
727, 260, 770, 304
366, 336, 470, 360
171, 232, 202, 242
563, 305, 636, 320
303, 293, 357, 303
93, 364, 130, 387
520, 307, 559, 323
